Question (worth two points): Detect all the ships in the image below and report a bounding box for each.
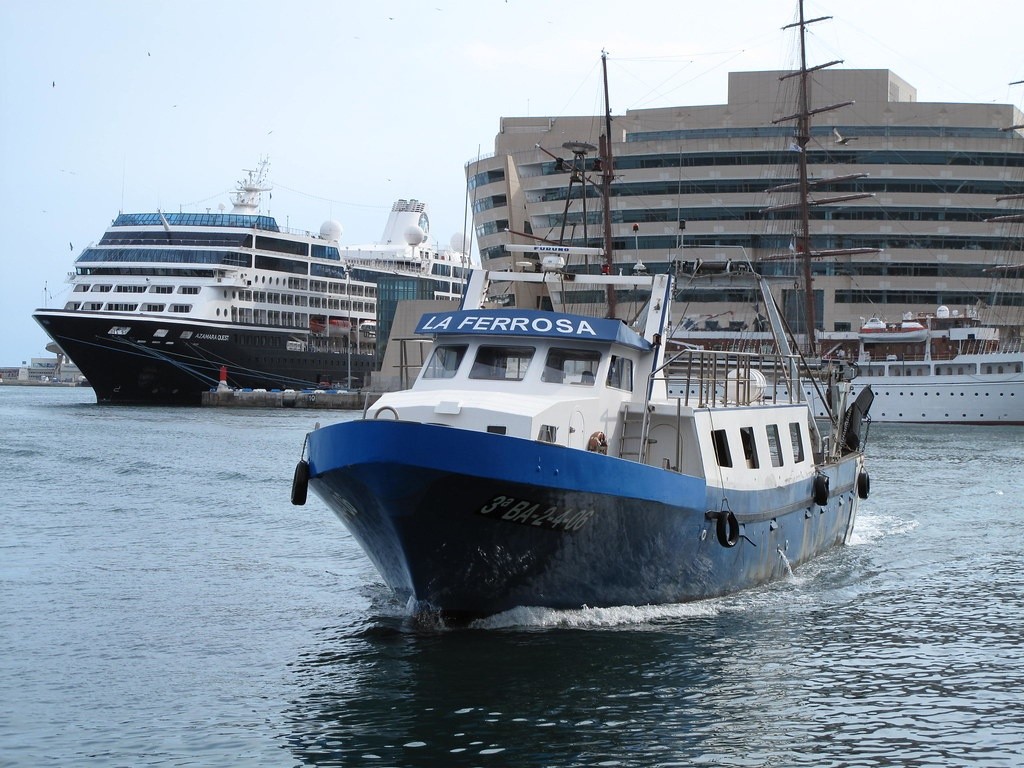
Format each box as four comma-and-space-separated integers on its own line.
30, 142, 475, 406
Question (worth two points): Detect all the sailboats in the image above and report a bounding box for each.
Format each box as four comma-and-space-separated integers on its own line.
583, 0, 1024, 430
286, 47, 879, 625
